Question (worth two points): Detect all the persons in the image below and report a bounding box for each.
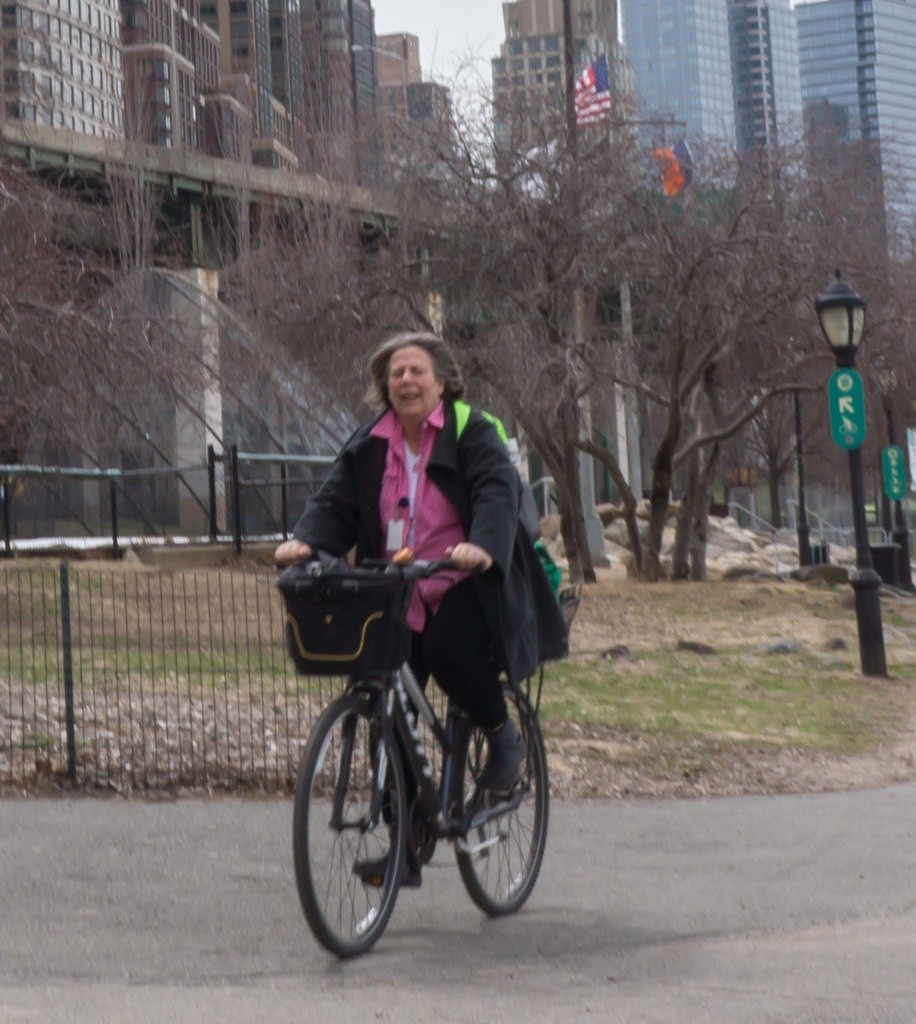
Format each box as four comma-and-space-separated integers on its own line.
273, 328, 570, 888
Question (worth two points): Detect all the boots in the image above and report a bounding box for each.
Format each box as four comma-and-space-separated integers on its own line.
479, 715, 528, 789
353, 848, 425, 888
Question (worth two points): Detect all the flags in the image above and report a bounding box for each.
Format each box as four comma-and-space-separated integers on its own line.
573, 52, 613, 126
648, 140, 696, 196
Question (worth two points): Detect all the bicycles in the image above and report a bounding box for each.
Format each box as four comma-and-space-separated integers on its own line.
272, 546, 568, 956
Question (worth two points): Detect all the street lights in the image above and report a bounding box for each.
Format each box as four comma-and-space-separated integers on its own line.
867, 348, 915, 594
814, 264, 886, 674
